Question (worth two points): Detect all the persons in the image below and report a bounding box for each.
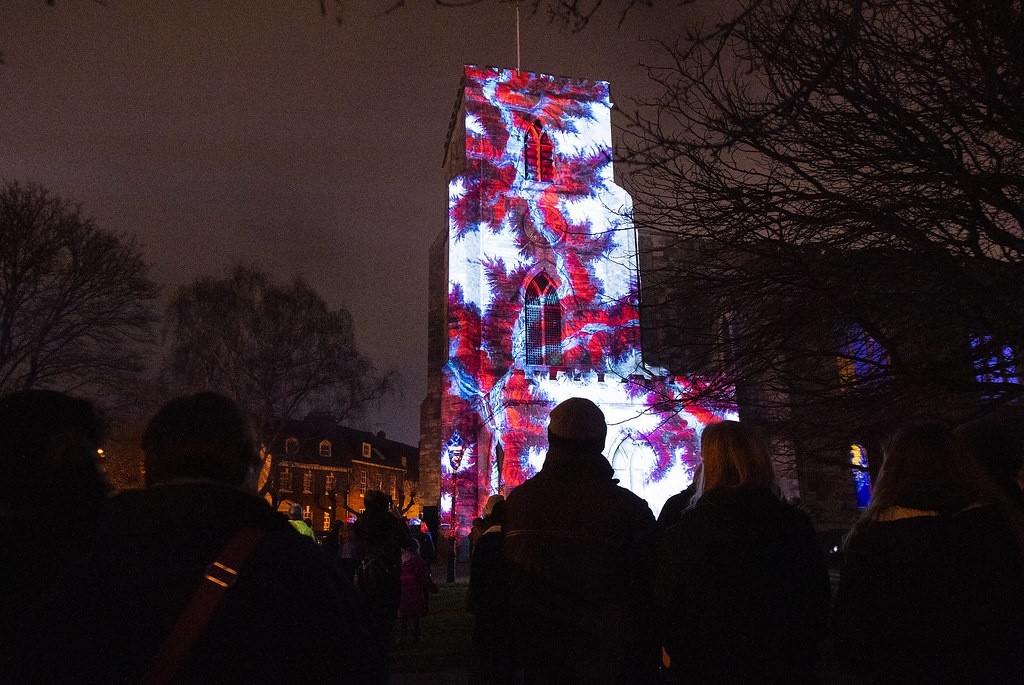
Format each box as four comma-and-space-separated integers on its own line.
814, 423, 1024, 684
641, 418, 834, 685
0, 391, 507, 684
496, 397, 661, 685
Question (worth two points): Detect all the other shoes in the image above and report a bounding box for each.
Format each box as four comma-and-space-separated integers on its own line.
397, 637, 410, 647
412, 636, 424, 645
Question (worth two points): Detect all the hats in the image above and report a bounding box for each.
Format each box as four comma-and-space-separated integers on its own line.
547, 398, 606, 453
482, 495, 504, 516
